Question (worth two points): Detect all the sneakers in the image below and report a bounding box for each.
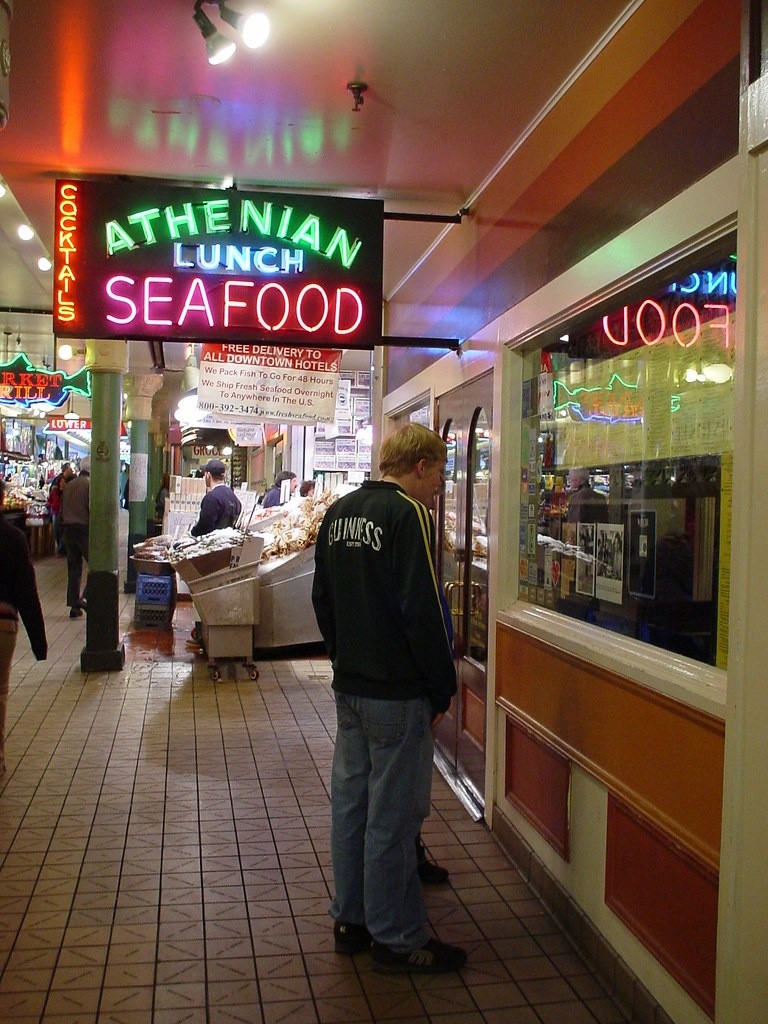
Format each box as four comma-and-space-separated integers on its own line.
370, 934, 468, 975
332, 916, 372, 957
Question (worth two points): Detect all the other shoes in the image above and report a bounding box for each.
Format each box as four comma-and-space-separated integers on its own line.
70, 607, 82, 618
77, 597, 87, 611
418, 845, 449, 884
186, 639, 201, 648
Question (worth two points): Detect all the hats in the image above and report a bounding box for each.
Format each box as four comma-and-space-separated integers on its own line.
199, 459, 225, 476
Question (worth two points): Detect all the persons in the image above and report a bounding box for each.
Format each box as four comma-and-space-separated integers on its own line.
300, 480, 315, 497
576, 527, 622, 594
262, 470, 298, 509
311, 422, 471, 976
403, 469, 456, 885
47, 476, 69, 559
61, 455, 91, 618
153, 472, 171, 523
53, 462, 78, 487
119, 462, 130, 510
189, 460, 242, 538
0, 479, 48, 780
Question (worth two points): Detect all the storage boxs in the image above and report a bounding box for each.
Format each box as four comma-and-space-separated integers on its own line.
190, 478, 194, 494
169, 493, 174, 510
133, 602, 176, 630
136, 572, 177, 605
198, 478, 202, 493
175, 476, 181, 494
180, 495, 185, 510
191, 494, 196, 511
186, 478, 189, 494
175, 495, 180, 510
203, 479, 206, 494
197, 494, 202, 510
194, 478, 198, 493
181, 478, 186, 494
185, 495, 191, 511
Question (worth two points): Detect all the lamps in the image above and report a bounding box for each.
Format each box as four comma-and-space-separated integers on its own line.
192, 0, 271, 66
223, 442, 232, 455
59, 344, 88, 361
64, 392, 80, 420
